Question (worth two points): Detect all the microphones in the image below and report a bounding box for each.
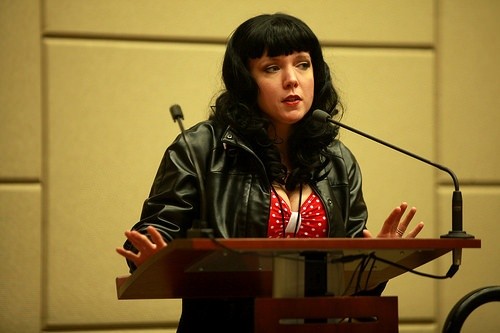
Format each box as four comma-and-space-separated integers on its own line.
312, 109, 475, 238
169, 104, 212, 238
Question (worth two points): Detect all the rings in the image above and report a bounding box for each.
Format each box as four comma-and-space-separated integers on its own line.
396, 228, 403, 236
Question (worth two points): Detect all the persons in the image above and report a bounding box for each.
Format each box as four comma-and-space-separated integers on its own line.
114, 13, 425, 333
